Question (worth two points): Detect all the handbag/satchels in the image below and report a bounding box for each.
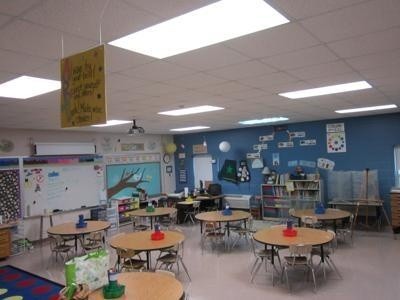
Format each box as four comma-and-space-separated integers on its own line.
63, 248, 110, 298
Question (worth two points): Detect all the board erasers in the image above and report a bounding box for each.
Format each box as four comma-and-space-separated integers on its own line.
81, 206, 85, 208
53, 209, 59, 212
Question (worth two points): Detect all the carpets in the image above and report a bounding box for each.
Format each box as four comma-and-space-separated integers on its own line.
1, 264, 65, 299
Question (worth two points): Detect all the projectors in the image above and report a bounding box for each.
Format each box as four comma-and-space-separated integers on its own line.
127, 127, 145, 135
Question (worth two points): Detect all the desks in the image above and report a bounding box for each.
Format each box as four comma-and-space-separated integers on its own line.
328, 199, 385, 232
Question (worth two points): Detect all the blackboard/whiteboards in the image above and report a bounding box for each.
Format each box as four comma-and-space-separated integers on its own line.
20, 156, 107, 219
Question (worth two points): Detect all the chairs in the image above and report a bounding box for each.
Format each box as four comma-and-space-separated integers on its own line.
47, 207, 191, 299
194, 210, 255, 253
247, 209, 354, 294
181, 200, 200, 225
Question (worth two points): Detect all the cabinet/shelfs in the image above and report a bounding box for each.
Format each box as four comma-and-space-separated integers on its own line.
111, 198, 139, 226
261, 180, 324, 220
390, 185, 400, 234
1, 228, 11, 260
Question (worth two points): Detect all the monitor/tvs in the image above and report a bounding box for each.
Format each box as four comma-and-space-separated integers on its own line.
208, 184, 221, 196
